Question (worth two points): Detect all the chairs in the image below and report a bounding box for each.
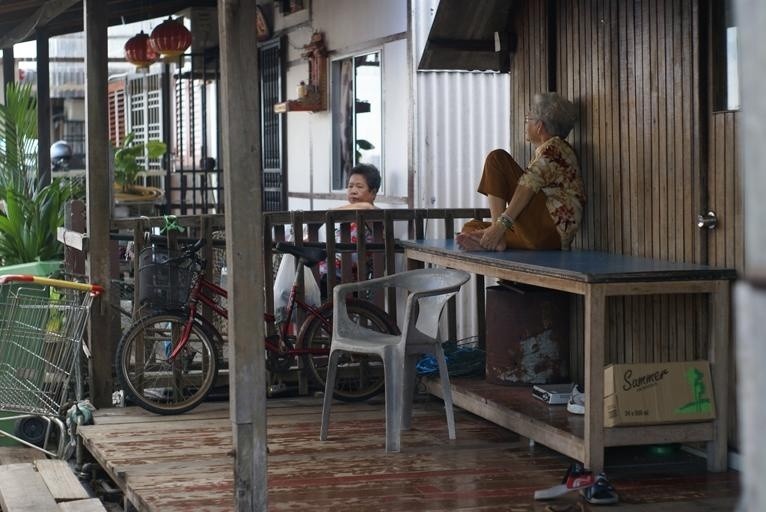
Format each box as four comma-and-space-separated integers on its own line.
315, 265, 476, 453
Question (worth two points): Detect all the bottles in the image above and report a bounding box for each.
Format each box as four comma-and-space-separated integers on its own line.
296, 80, 305, 98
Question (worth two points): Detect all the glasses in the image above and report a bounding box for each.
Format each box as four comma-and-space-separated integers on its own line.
524, 114, 537, 122
52, 155, 70, 162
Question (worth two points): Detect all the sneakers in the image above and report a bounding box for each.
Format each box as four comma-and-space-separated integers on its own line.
566, 384, 585, 415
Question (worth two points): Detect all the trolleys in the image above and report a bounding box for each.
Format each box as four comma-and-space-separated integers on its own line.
0, 271, 104, 491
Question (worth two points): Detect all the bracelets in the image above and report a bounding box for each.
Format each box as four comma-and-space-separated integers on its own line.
497, 212, 515, 229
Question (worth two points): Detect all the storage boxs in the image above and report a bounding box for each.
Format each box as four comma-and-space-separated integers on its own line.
602, 358, 717, 430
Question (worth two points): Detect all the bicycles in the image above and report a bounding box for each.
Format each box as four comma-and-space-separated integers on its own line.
114, 232, 400, 415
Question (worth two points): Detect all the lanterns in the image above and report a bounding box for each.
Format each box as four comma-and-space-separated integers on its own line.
123, 31, 160, 75
149, 17, 192, 68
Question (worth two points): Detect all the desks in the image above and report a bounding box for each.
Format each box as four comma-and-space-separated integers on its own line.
396, 233, 736, 476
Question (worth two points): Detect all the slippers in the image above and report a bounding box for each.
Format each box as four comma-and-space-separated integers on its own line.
534, 462, 599, 500
580, 467, 619, 506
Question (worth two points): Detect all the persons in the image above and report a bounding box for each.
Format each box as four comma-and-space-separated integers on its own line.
453, 90, 586, 254
265, 162, 386, 344
